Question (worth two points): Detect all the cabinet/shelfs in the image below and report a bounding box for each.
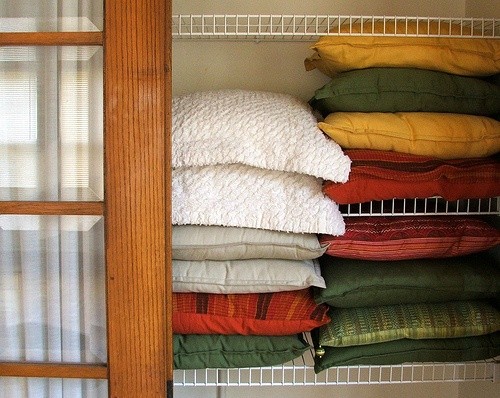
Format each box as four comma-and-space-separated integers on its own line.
170, 14, 500, 387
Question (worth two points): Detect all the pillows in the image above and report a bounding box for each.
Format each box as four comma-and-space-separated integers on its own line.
171, 21, 500, 374
